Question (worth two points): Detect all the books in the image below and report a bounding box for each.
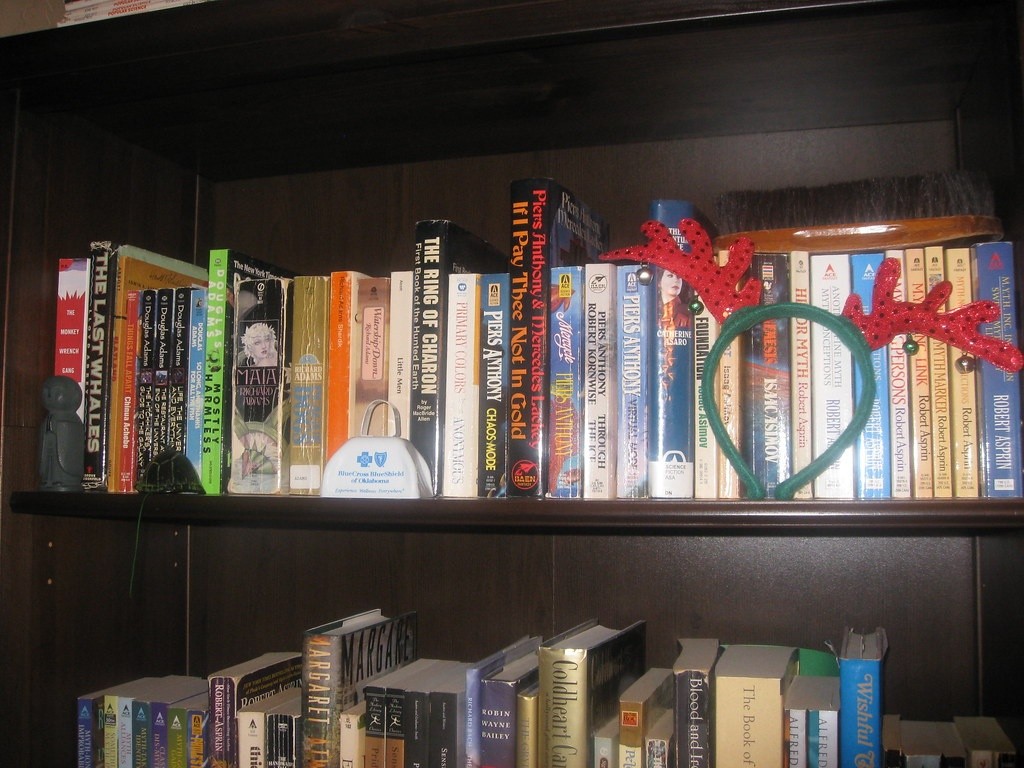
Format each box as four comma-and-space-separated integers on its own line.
53, 175, 1024, 504
77, 609, 1014, 768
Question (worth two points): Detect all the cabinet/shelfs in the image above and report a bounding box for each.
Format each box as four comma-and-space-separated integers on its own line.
0, 0, 1024, 768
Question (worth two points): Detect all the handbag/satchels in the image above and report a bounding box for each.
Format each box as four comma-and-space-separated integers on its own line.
320, 399, 434, 499
